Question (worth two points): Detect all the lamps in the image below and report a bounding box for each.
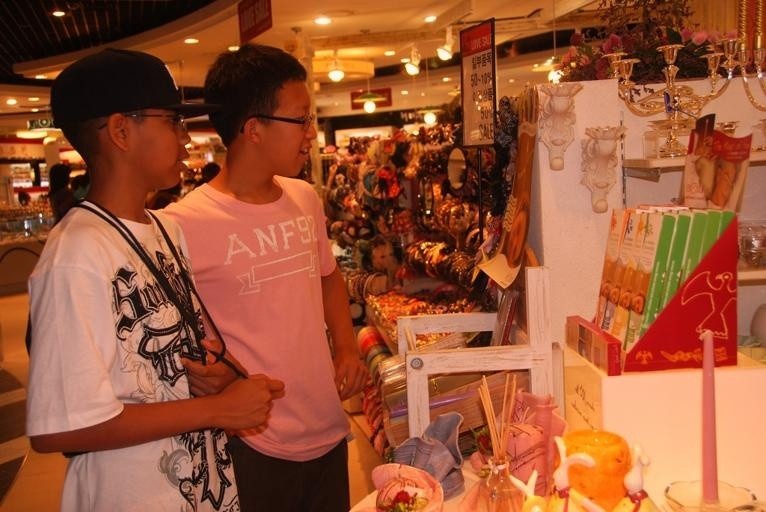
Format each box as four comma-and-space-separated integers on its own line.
310, 60, 375, 82
352, 77, 387, 114
327, 50, 345, 82
436, 26, 458, 61
405, 46, 422, 75
418, 52, 442, 124
532, 0, 563, 83
602, 0, 766, 158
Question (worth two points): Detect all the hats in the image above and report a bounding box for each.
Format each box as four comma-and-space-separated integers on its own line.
49, 46, 223, 129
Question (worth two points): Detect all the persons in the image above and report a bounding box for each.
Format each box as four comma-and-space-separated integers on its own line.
599, 279, 612, 298
23, 47, 285, 512
159, 43, 371, 511
609, 283, 621, 304
619, 287, 632, 310
49, 159, 222, 224
630, 292, 646, 315
710, 157, 742, 207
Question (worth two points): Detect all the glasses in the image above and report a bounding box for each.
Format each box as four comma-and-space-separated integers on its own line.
240, 111, 317, 134
97, 114, 185, 130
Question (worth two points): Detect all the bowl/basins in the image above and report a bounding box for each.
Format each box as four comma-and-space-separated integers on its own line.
737, 219, 766, 268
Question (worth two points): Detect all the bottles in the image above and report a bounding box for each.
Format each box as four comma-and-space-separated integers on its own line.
564, 431, 634, 502
13, 167, 48, 179
475, 454, 525, 512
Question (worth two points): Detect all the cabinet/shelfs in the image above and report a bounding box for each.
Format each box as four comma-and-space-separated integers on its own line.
621, 150, 766, 371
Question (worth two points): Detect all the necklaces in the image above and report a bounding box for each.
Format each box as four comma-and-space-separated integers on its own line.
71, 197, 245, 380
